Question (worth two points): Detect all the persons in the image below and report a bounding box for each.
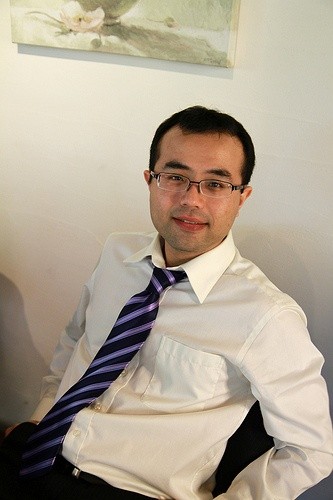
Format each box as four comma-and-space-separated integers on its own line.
0, 104, 333, 500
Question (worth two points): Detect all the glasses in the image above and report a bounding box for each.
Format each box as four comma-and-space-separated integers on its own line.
149, 170, 247, 199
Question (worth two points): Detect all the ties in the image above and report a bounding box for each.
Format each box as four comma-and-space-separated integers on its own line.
18, 256, 190, 484
